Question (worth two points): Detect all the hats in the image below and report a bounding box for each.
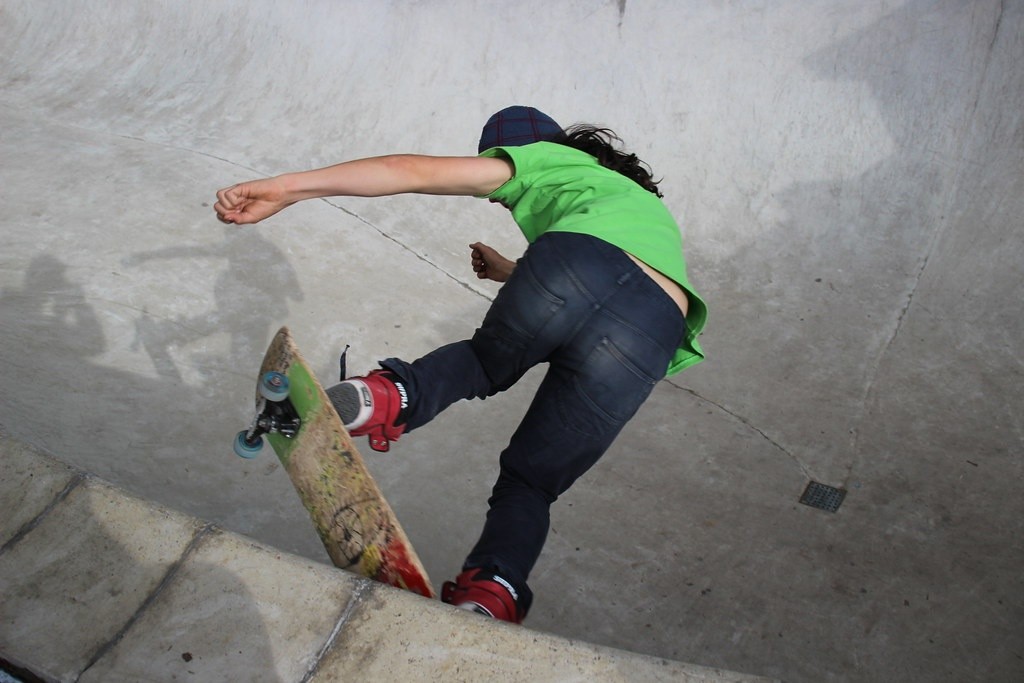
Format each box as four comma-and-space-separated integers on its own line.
478, 105, 567, 155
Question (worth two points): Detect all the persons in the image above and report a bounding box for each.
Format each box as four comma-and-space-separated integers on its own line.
216, 105, 707, 625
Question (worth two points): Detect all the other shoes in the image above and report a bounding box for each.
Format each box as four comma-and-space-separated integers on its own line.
441, 568, 526, 628
324, 345, 407, 452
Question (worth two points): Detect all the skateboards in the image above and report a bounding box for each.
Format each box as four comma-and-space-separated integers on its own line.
231, 325, 443, 604
